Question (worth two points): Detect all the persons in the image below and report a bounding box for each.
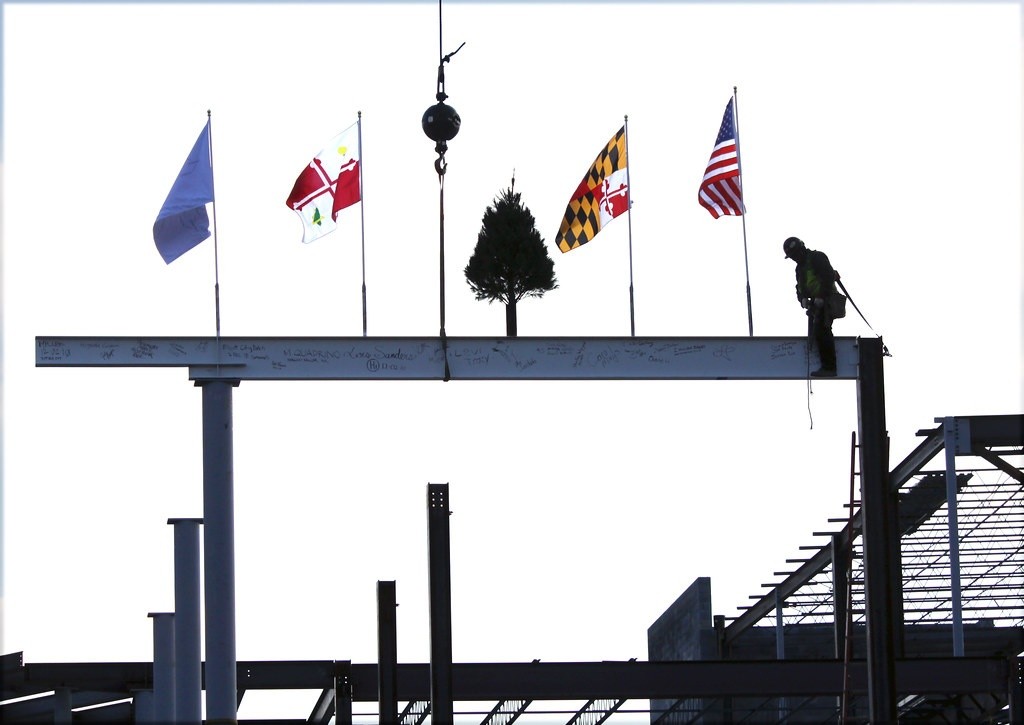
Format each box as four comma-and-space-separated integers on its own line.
781, 237, 839, 378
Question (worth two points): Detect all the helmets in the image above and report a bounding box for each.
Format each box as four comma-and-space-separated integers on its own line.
783, 237, 804, 259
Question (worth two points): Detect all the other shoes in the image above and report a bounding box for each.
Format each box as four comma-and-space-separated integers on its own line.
810, 368, 837, 376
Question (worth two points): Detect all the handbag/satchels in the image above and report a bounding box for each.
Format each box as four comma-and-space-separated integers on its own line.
834, 294, 847, 318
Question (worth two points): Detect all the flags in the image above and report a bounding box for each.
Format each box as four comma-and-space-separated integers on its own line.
153, 121, 213, 266
286, 121, 361, 244
555, 126, 629, 254
698, 96, 747, 219
